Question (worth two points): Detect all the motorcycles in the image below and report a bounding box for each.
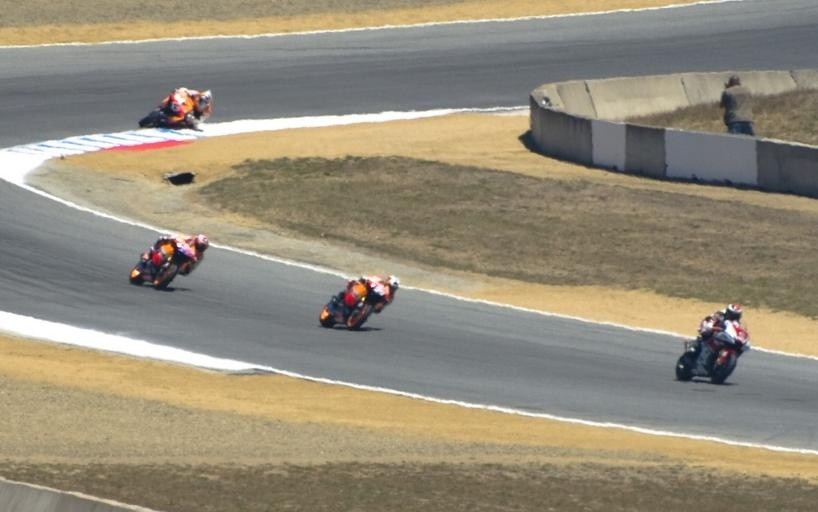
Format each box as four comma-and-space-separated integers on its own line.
139, 88, 214, 129
319, 278, 393, 330
676, 316, 752, 385
130, 234, 198, 291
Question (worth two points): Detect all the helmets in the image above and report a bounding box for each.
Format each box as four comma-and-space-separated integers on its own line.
385, 274, 401, 290
197, 89, 212, 108
727, 302, 743, 321
194, 232, 210, 252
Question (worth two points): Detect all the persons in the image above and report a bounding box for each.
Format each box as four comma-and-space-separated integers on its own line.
721, 75, 754, 136
185, 89, 214, 127
176, 233, 208, 275
708, 302, 743, 324
364, 274, 399, 313
